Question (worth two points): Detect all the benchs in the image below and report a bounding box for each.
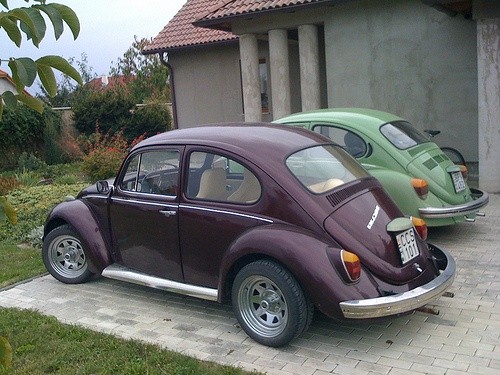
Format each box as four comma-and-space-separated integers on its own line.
344, 132, 366, 156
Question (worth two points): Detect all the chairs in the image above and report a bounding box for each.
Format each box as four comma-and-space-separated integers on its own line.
196, 168, 226, 201
226, 168, 261, 203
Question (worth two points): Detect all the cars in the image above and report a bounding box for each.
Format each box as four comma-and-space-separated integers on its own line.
42, 120, 458, 347
212, 107, 489, 228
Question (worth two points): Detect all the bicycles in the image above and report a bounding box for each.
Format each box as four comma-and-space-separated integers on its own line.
388, 129, 469, 182
